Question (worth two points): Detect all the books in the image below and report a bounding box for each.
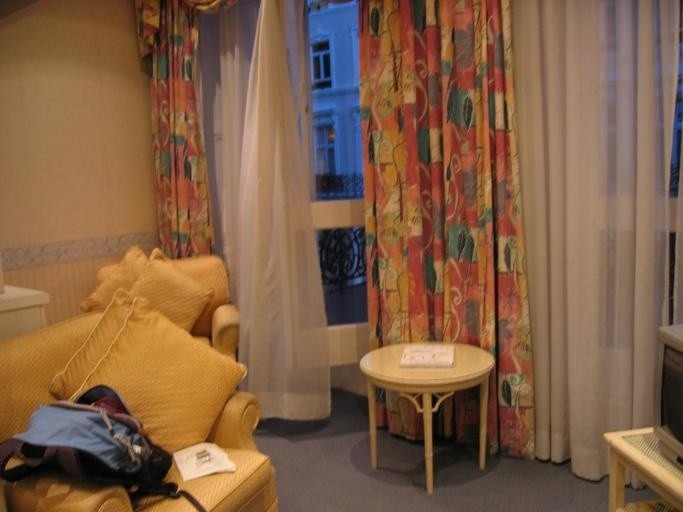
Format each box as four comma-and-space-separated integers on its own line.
171, 440, 235, 481
400, 342, 456, 367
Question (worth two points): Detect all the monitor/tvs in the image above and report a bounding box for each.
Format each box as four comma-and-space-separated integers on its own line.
653, 325, 683, 469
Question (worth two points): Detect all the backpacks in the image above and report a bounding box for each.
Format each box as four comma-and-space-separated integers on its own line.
1, 386, 178, 501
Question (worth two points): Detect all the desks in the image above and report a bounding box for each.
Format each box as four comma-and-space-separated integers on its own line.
603, 427, 683, 512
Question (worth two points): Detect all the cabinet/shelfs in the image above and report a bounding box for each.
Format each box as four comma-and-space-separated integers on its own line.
0, 284, 50, 342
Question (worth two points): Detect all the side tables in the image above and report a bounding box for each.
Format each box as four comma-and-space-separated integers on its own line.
358, 343, 504, 493
